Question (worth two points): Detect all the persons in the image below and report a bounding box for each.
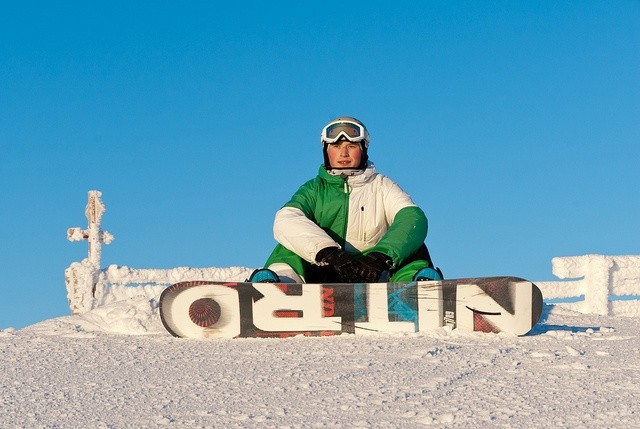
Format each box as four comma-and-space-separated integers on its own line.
249, 117, 443, 282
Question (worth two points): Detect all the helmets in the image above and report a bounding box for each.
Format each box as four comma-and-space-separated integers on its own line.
321, 117, 370, 176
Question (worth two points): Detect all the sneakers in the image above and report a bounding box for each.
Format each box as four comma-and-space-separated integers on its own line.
413, 266, 444, 281
246, 269, 279, 282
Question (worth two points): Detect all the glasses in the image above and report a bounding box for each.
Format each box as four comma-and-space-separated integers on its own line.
321, 120, 369, 145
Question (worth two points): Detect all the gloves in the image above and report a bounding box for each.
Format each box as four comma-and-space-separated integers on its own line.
349, 252, 392, 282
322, 248, 353, 282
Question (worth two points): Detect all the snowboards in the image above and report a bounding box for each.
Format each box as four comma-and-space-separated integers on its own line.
159, 276, 543, 339
